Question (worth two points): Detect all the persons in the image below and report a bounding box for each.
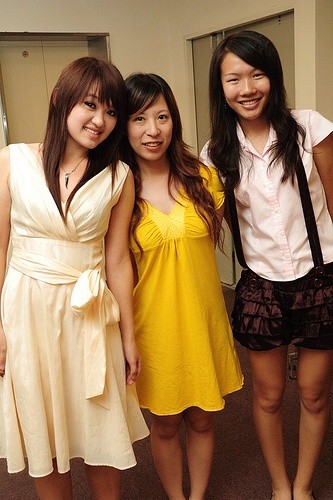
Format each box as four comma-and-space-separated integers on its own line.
122, 73, 244, 500
201, 31, 333, 500
0, 56, 150, 500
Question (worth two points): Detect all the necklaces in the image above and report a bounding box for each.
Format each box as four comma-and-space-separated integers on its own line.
60, 156, 87, 189
